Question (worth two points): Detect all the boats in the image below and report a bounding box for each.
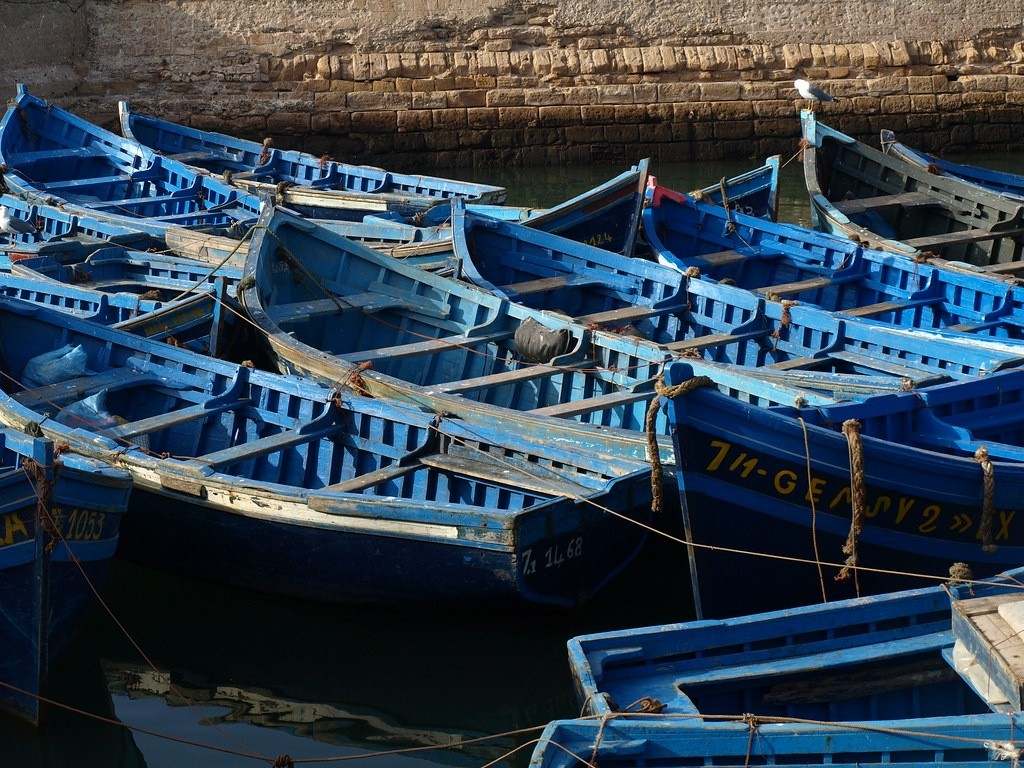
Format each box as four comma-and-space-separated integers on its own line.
566, 568, 1021, 734
2, 423, 133, 724
2, 80, 1023, 628
530, 712, 1021, 768
